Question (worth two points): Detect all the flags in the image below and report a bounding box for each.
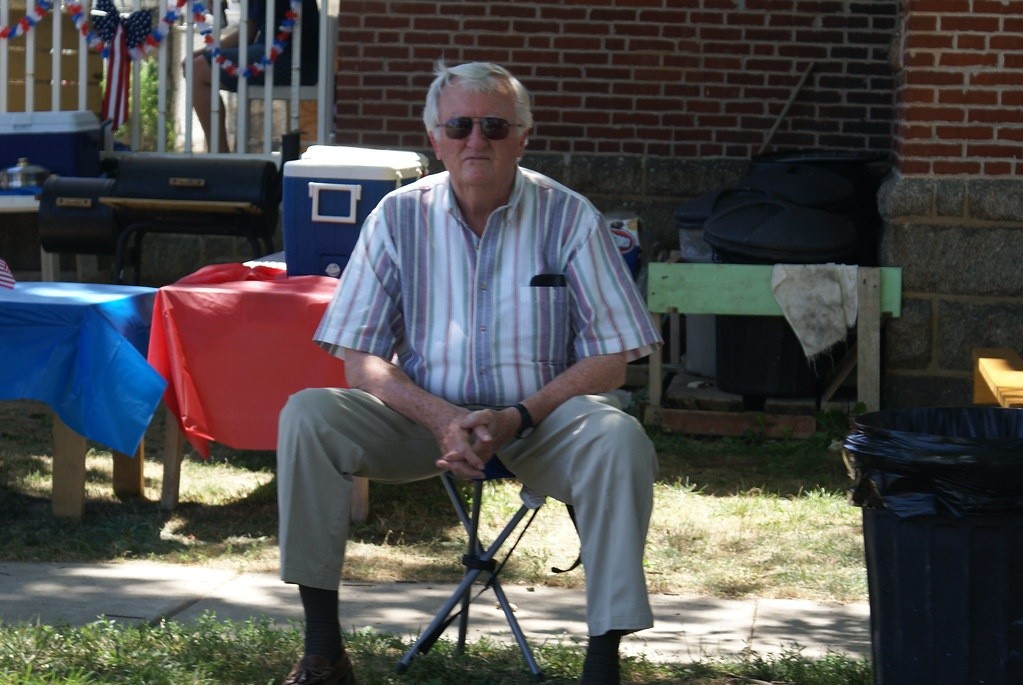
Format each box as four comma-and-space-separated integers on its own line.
0, 259, 16, 289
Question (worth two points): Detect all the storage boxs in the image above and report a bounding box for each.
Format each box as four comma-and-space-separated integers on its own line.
0, 110, 102, 178
283, 144, 430, 279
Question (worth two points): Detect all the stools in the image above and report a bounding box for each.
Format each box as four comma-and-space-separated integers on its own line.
394, 454, 581, 683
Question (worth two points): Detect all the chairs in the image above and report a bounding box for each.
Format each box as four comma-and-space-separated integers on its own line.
236, 15, 336, 154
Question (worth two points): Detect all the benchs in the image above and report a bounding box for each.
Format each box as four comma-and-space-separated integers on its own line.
967, 344, 1023, 409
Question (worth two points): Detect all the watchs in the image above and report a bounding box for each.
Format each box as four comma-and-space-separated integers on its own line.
513, 404, 536, 439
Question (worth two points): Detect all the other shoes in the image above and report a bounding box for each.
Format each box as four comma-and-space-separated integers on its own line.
283, 651, 350, 685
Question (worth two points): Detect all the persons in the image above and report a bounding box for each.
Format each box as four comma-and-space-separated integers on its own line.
179, 0, 319, 153
276, 61, 664, 685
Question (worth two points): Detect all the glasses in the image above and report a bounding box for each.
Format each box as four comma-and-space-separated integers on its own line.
433, 116, 524, 140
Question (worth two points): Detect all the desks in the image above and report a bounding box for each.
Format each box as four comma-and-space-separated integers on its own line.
147, 263, 370, 522
0, 281, 158, 525
98, 199, 282, 285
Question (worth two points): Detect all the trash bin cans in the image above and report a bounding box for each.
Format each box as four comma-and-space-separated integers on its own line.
847, 402, 1022, 685
